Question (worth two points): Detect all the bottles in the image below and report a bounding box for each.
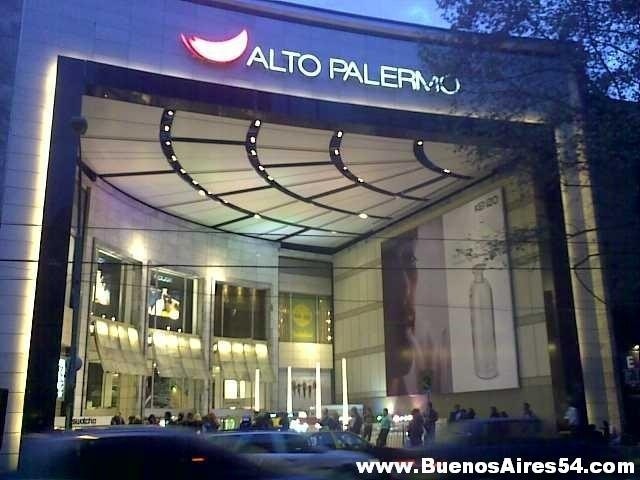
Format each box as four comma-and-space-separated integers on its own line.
469, 264, 498, 379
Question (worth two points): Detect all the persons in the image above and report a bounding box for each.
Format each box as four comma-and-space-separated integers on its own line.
383, 231, 425, 394
112, 404, 611, 448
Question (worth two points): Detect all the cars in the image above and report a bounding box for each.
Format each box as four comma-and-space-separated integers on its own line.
1, 416, 626, 480
